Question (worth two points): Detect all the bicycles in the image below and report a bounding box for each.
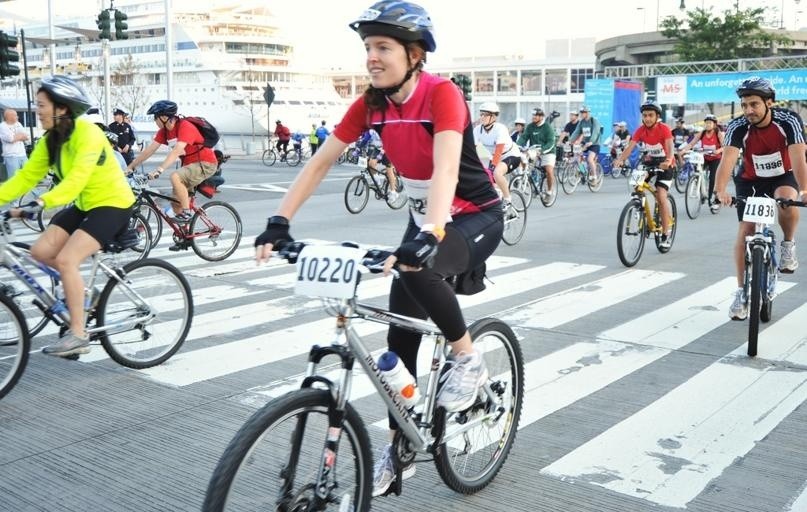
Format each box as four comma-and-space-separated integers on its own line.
262, 139, 301, 167
121, 169, 242, 261
287, 137, 366, 165
0, 210, 194, 400
0, 134, 165, 345
491, 164, 527, 246
507, 141, 728, 221
712, 196, 807, 357
344, 151, 407, 214
200, 235, 524, 511
610, 164, 677, 268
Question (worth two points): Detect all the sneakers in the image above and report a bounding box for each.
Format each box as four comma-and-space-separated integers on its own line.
777, 238, 798, 273
501, 197, 511, 212
656, 233, 671, 249
728, 288, 747, 321
590, 176, 596, 186
43, 329, 90, 356
543, 189, 552, 203
169, 238, 190, 250
387, 193, 398, 204
370, 443, 416, 497
710, 199, 720, 210
435, 351, 489, 411
171, 213, 194, 224
86, 286, 101, 310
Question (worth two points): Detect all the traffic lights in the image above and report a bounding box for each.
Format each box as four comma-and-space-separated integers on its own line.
97, 11, 110, 40
0, 30, 20, 80
450, 74, 463, 90
462, 76, 471, 103
114, 11, 128, 40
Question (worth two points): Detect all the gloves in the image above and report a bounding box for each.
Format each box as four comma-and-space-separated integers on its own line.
7, 200, 42, 223
254, 215, 294, 250
392, 230, 440, 269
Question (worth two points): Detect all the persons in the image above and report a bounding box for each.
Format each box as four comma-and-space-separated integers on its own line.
252, 0, 505, 498
272, 75, 806, 321
1, 75, 229, 355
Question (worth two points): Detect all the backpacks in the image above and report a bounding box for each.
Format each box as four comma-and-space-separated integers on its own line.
164, 114, 220, 157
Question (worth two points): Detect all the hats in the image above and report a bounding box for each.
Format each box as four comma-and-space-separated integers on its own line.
112, 102, 129, 116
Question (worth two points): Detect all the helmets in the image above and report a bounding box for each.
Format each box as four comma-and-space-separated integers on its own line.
478, 102, 499, 116
147, 100, 177, 116
40, 74, 92, 119
736, 77, 775, 103
513, 105, 590, 125
675, 117, 684, 122
703, 115, 716, 122
348, 0, 435, 52
639, 99, 662, 114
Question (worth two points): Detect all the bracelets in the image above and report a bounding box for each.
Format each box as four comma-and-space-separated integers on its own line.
419, 225, 445, 243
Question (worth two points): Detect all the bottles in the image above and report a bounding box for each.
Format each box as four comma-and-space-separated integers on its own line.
377, 353, 421, 410
53, 279, 66, 302
164, 205, 175, 219
